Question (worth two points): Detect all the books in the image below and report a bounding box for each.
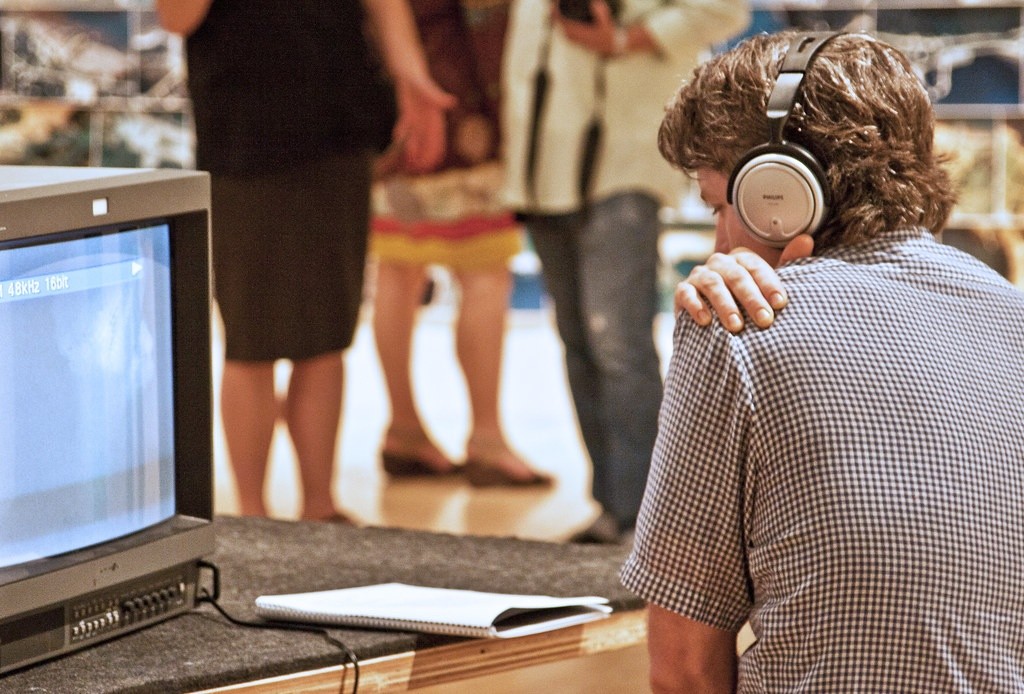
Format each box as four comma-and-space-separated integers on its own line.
253, 582, 615, 642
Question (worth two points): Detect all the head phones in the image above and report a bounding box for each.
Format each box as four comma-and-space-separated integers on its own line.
727, 32, 848, 247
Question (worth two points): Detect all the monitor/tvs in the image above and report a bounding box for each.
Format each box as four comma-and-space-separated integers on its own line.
2, 163, 215, 625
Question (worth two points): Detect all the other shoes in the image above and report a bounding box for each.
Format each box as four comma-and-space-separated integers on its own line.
565, 514, 638, 546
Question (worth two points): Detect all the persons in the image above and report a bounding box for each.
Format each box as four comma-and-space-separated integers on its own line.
618, 29, 1024, 686
149, 1, 459, 530
371, 1, 552, 493
498, 4, 756, 549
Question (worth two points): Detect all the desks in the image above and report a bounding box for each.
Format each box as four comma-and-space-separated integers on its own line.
0, 513, 757, 694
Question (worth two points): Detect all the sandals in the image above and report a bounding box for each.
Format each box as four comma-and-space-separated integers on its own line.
383, 426, 461, 478
466, 436, 553, 489
